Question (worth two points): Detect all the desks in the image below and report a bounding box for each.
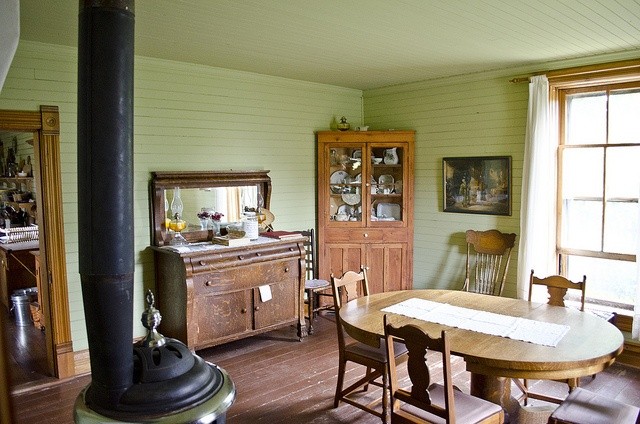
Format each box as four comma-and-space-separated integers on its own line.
339, 289, 624, 418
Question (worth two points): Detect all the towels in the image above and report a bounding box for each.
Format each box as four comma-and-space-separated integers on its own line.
381, 297, 571, 348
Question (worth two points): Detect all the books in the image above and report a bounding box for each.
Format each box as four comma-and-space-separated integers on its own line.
212, 230, 251, 248
259, 231, 305, 242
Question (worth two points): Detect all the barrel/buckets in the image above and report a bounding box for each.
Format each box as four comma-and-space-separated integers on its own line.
12, 288, 35, 325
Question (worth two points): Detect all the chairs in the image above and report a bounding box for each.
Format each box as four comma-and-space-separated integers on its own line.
293, 229, 333, 333
460, 229, 517, 297
383, 314, 504, 424
515, 269, 588, 405
330, 264, 408, 424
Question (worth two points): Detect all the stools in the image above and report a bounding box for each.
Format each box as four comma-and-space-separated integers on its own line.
547, 386, 640, 424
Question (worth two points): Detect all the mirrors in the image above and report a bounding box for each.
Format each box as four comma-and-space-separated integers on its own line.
149, 169, 274, 244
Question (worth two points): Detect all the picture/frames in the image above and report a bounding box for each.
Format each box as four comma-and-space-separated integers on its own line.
441, 157, 513, 216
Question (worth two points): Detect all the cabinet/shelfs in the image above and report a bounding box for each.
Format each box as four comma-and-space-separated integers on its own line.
151, 231, 309, 355
315, 130, 414, 317
